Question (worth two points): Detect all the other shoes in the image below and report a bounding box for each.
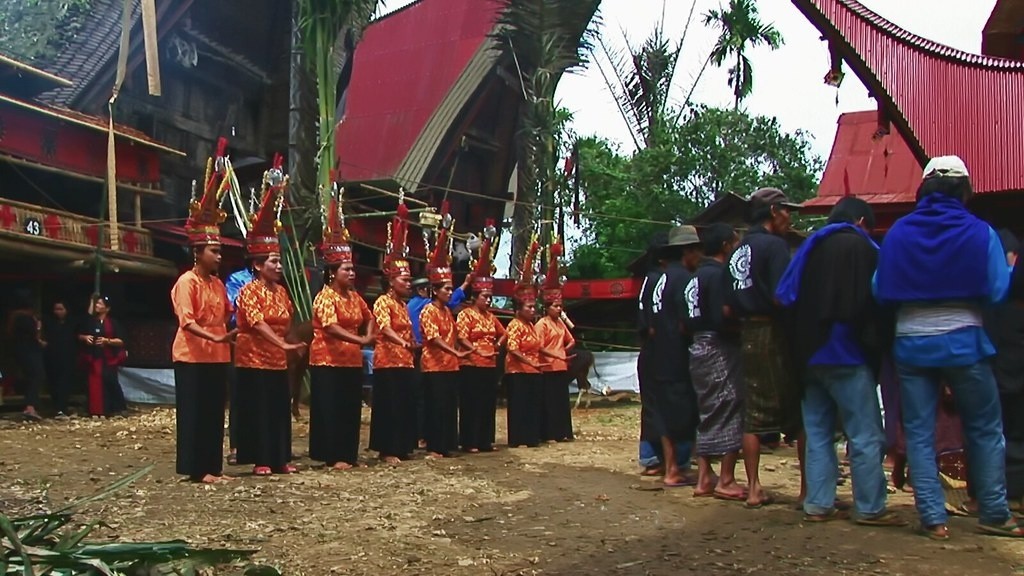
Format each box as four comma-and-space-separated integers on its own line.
22, 409, 42, 421
54, 411, 64, 419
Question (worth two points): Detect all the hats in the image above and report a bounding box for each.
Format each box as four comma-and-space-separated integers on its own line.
244, 154, 286, 255
751, 187, 804, 209
183, 138, 230, 243
425, 201, 455, 283
469, 218, 496, 292
318, 183, 355, 265
541, 243, 564, 302
665, 225, 700, 246
921, 154, 969, 179
514, 242, 536, 302
382, 186, 412, 277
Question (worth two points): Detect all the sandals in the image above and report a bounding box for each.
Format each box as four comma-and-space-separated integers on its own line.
273, 465, 299, 473
254, 465, 272, 475
227, 453, 237, 464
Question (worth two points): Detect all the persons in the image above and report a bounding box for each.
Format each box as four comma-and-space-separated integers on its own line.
171, 223, 575, 484
7, 298, 47, 421
78, 293, 127, 415
636, 153, 1024, 542
49, 302, 77, 415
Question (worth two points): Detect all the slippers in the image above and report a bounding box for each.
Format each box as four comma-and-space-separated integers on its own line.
692, 488, 714, 497
647, 468, 666, 476
803, 509, 850, 520
712, 491, 747, 500
744, 495, 775, 508
858, 514, 909, 526
915, 526, 950, 540
665, 475, 699, 486
975, 520, 1024, 536
